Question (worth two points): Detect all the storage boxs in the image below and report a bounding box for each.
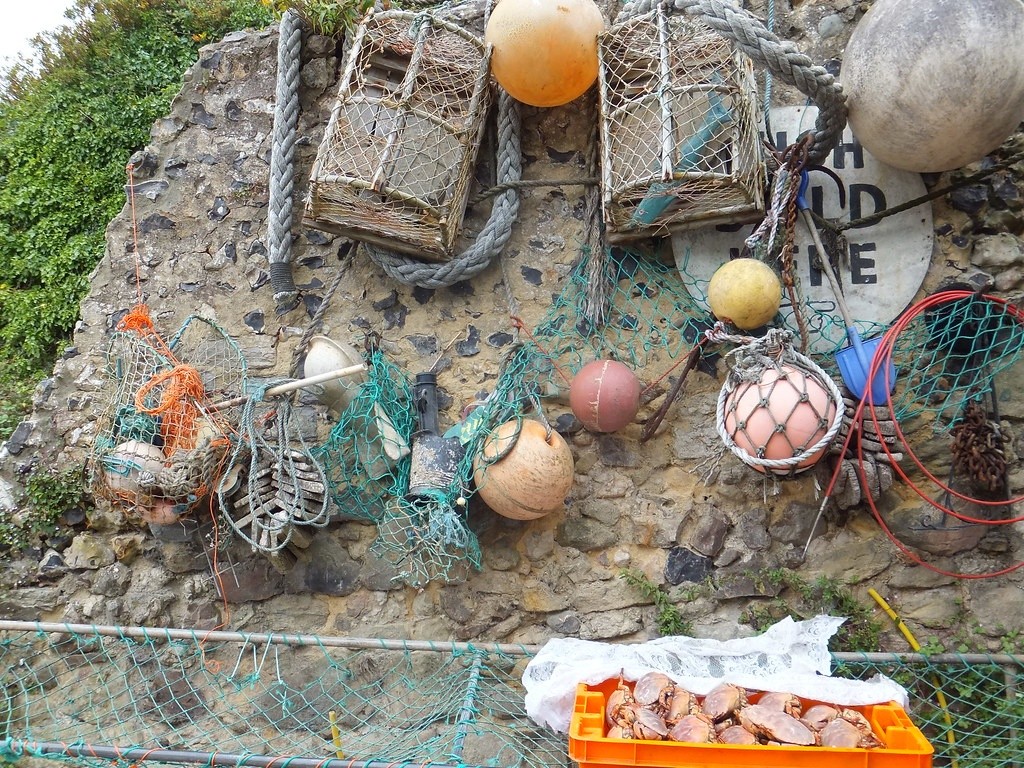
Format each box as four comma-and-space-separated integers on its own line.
568, 676, 934, 768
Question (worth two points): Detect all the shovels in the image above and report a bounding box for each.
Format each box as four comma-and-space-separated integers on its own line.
796, 167, 897, 407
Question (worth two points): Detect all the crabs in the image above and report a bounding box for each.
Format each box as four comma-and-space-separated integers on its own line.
606, 668, 885, 748
